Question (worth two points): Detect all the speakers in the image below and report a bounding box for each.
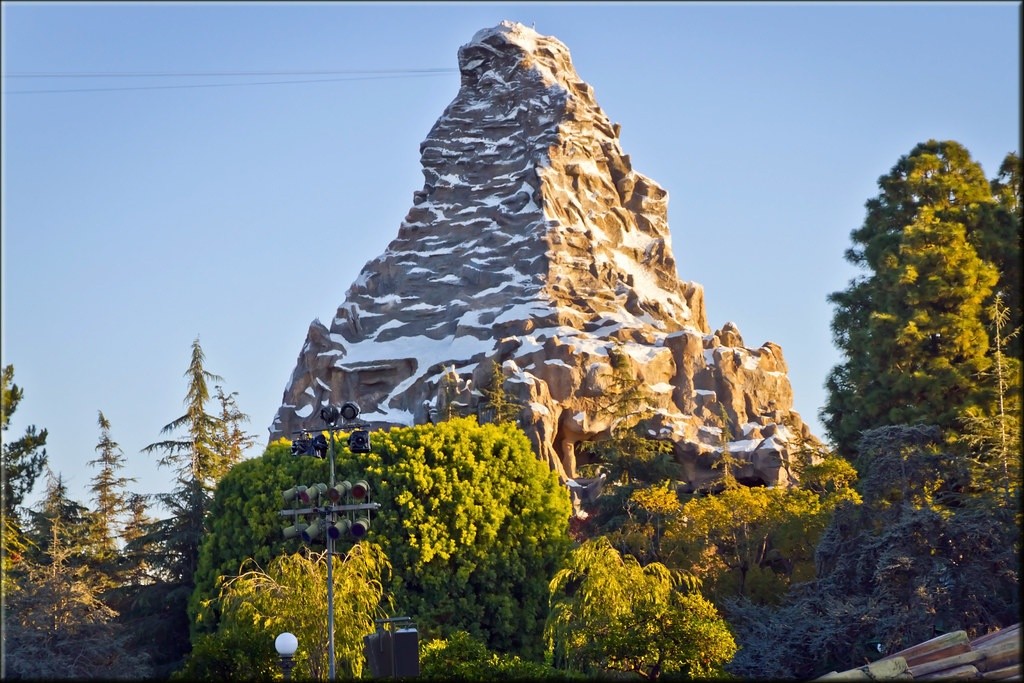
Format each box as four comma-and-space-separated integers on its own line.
363, 631, 392, 679
392, 628, 420, 678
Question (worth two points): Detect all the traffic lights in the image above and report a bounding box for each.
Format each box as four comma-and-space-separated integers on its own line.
392, 625, 421, 678
362, 630, 393, 679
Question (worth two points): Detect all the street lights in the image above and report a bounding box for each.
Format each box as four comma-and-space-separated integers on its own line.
274, 632, 299, 683
276, 399, 383, 683
